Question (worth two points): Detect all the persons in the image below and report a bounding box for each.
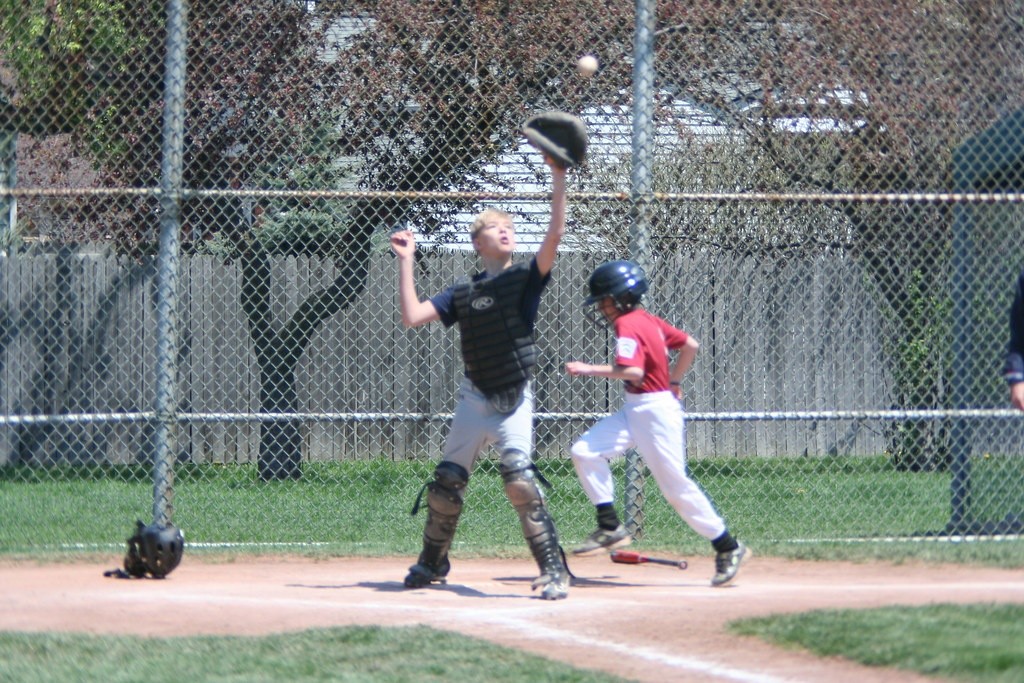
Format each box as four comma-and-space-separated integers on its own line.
565, 259, 752, 586
1004, 265, 1024, 411
390, 111, 575, 602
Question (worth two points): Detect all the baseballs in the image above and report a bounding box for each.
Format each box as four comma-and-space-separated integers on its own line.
575, 55, 598, 77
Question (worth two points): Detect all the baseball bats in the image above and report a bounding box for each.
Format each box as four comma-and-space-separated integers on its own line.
609, 547, 687, 571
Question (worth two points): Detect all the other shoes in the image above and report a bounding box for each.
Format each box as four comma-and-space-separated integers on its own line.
404, 559, 449, 587
542, 569, 571, 600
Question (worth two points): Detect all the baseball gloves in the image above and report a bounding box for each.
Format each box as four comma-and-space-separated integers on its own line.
521, 110, 587, 171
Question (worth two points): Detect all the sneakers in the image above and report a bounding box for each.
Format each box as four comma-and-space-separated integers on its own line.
712, 539, 752, 586
573, 524, 632, 557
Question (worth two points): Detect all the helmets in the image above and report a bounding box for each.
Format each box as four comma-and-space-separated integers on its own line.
581, 261, 648, 329
